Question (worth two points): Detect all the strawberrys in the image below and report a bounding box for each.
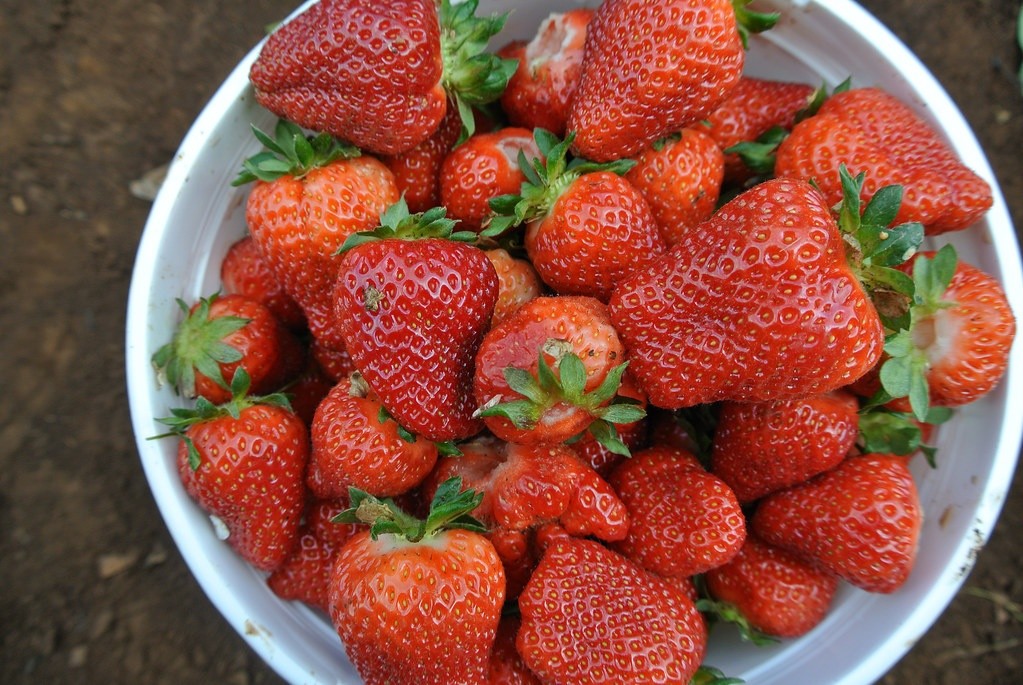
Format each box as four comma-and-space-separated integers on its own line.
144, 0, 1015, 685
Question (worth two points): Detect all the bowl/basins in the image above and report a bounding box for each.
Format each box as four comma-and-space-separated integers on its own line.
125, 0, 1023, 684
